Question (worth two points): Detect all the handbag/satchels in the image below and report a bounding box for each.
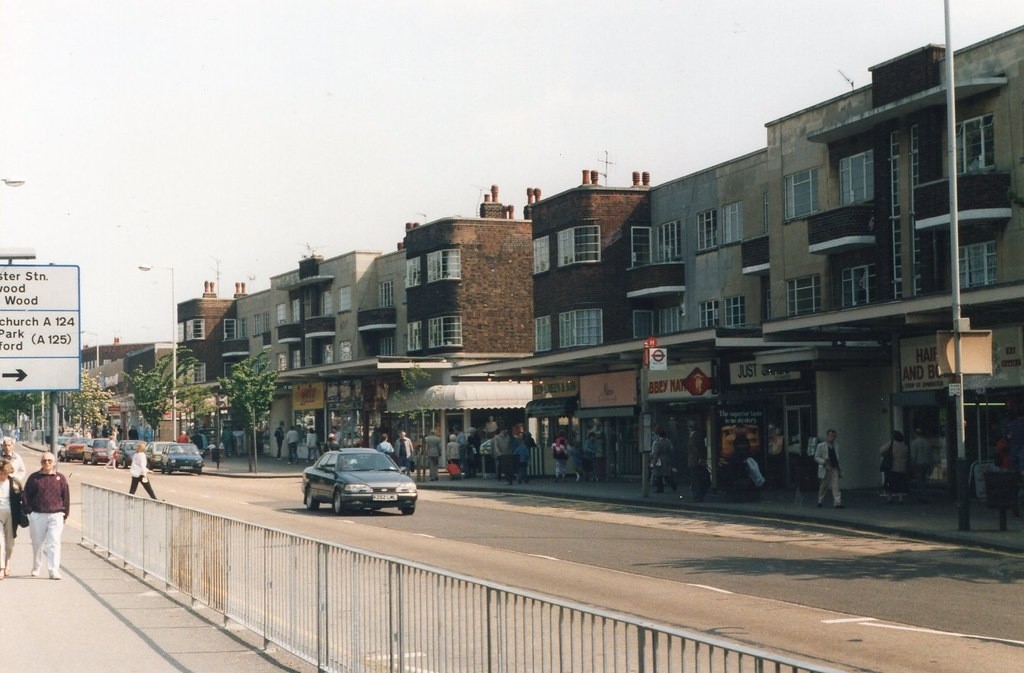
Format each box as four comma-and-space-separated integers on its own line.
410, 460, 415, 472
880, 440, 892, 471
10, 478, 29, 527
114, 451, 118, 459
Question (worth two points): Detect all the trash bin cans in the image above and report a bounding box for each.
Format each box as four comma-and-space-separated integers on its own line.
497, 454, 519, 476
984, 472, 1020, 509
212, 448, 219, 462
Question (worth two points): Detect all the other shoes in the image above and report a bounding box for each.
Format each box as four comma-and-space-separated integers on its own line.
104, 466, 107, 471
817, 502, 822, 507
288, 462, 290, 464
0, 570, 5, 579
5, 569, 9, 576
32, 570, 39, 578
835, 505, 847, 510
277, 458, 282, 460
49, 572, 61, 580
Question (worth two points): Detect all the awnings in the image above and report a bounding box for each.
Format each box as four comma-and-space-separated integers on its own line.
386, 385, 533, 410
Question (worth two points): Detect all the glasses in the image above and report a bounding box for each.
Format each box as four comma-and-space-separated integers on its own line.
41, 459, 54, 464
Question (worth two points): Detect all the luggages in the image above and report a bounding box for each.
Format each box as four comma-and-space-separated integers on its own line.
447, 461, 461, 480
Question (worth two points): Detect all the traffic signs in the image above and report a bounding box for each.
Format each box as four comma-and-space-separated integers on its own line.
0, 264, 81, 392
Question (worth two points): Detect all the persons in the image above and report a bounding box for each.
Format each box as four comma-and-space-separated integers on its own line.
687, 421, 704, 469
21, 452, 71, 580
909, 428, 933, 505
551, 431, 587, 482
583, 431, 601, 482
648, 424, 676, 493
91, 423, 138, 440
425, 430, 441, 481
395, 431, 413, 475
324, 427, 341, 451
285, 426, 299, 465
274, 421, 285, 460
377, 433, 394, 454
0, 427, 26, 579
128, 443, 158, 499
991, 430, 1010, 470
814, 430, 846, 509
305, 428, 322, 463
447, 423, 534, 485
180, 431, 208, 458
879, 430, 907, 503
222, 427, 234, 457
104, 436, 118, 470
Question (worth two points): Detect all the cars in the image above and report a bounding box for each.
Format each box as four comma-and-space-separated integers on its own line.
56, 435, 205, 475
302, 448, 419, 516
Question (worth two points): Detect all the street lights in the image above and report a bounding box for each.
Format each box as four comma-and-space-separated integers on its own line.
137, 263, 177, 443
